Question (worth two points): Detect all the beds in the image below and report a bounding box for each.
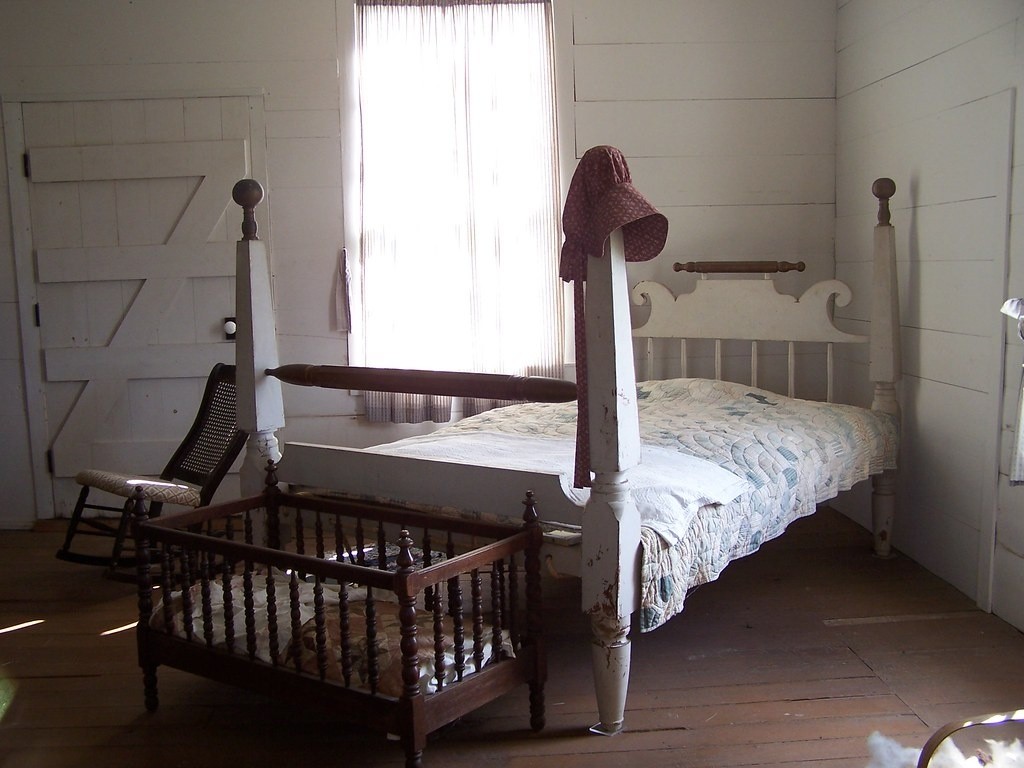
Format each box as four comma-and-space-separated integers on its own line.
128, 459, 545, 768
232, 146, 896, 724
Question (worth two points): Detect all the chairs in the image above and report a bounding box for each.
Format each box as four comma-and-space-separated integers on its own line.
55, 362, 247, 586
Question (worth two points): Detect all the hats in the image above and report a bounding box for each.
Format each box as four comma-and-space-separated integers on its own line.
559, 146, 669, 489
